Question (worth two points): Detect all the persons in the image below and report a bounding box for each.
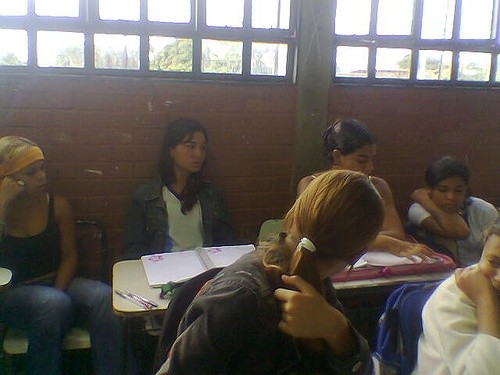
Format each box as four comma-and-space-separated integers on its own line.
295, 119, 437, 346
406, 154, 499, 271
408, 222, 500, 375
153, 169, 387, 375
120, 115, 241, 375
0, 135, 128, 375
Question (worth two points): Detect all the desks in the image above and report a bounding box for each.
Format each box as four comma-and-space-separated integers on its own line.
331, 270, 455, 290
112, 258, 174, 374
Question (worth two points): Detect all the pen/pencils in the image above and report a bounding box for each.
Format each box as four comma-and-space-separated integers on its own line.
115, 288, 158, 309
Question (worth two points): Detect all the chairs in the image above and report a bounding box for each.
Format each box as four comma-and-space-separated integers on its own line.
153, 267, 227, 374
373, 280, 445, 375
2, 220, 107, 355
